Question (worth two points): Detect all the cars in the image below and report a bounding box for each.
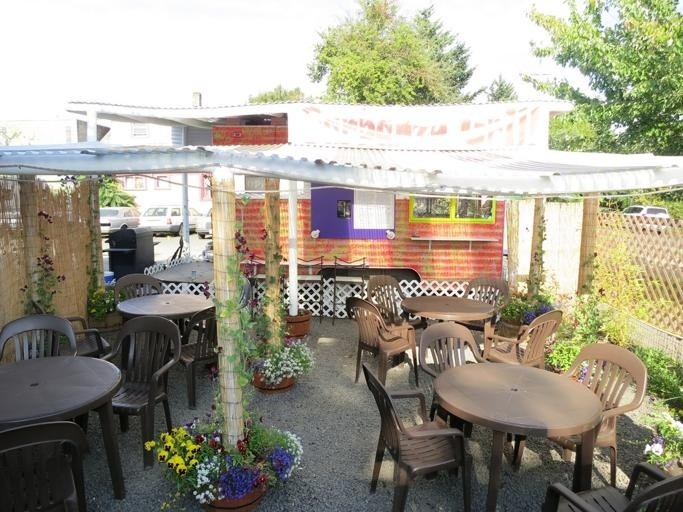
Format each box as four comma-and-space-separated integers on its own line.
1, 206, 214, 242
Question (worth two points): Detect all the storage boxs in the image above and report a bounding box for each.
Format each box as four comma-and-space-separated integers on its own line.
209, 123, 288, 146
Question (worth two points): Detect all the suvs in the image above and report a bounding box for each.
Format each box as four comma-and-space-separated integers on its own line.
621, 204, 670, 237
